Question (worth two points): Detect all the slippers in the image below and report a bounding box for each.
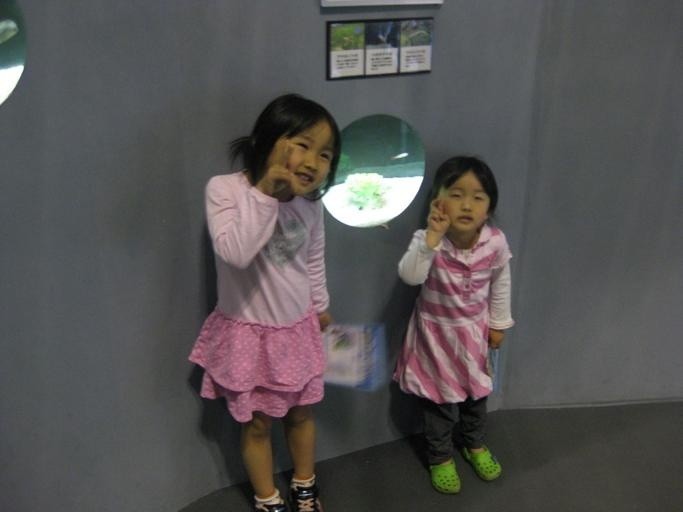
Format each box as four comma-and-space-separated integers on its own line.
461, 446, 501, 482
430, 461, 462, 493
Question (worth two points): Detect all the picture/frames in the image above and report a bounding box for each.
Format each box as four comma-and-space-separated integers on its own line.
325, 16, 433, 81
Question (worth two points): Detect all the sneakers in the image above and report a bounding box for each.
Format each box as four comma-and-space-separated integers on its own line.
292, 488, 324, 512
256, 500, 287, 511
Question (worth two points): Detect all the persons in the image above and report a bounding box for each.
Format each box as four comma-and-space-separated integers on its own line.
393, 157, 516, 493
184, 94, 340, 512
